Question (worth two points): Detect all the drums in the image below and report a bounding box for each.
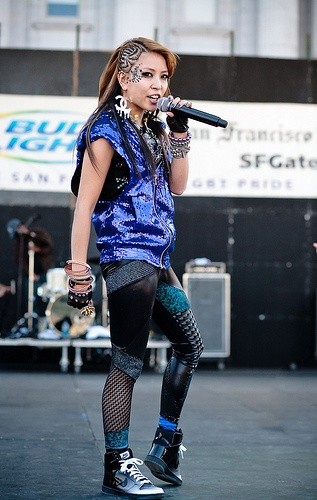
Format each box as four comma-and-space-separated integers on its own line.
45, 292, 96, 338
44, 264, 95, 291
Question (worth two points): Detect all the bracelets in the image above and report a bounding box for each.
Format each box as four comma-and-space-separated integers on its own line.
168, 130, 192, 159
64, 260, 96, 294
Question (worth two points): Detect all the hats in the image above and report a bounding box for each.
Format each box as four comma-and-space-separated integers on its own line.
8, 219, 20, 239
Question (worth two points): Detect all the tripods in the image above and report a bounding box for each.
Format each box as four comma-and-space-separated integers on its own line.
10, 235, 48, 336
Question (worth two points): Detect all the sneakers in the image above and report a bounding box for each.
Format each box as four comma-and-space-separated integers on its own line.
102, 449, 164, 500
144, 426, 185, 485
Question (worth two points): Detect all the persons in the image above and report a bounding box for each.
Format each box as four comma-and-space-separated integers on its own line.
7, 218, 53, 338
66, 38, 203, 500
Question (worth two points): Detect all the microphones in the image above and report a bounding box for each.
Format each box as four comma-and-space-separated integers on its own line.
156, 97, 228, 128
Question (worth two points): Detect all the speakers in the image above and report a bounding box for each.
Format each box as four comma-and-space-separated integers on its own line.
184, 272, 232, 357
